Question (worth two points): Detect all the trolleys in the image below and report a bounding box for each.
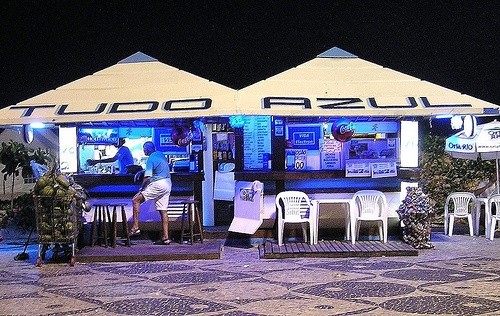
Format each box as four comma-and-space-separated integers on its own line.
32, 194, 81, 267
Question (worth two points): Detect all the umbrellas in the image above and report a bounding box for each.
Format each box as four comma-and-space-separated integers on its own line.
445, 119, 500, 194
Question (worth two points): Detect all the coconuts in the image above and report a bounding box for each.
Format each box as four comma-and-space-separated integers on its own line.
35, 175, 91, 239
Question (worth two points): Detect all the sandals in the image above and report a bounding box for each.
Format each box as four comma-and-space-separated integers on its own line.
125, 228, 141, 237
153, 239, 170, 245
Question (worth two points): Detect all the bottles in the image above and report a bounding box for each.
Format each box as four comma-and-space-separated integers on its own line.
212, 118, 232, 132
213, 141, 233, 160
268, 157, 271, 169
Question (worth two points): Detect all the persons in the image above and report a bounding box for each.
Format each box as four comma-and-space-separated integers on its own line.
128, 141, 172, 245
88, 138, 134, 176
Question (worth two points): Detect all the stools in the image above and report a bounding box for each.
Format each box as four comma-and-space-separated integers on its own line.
181, 201, 203, 244
91, 203, 131, 248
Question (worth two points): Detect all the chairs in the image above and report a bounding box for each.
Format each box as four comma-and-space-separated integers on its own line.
352, 190, 389, 243
276, 191, 313, 247
486, 194, 500, 240
444, 192, 476, 236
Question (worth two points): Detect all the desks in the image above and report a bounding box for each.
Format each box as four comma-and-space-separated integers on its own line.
473, 197, 500, 239
312, 199, 359, 245
